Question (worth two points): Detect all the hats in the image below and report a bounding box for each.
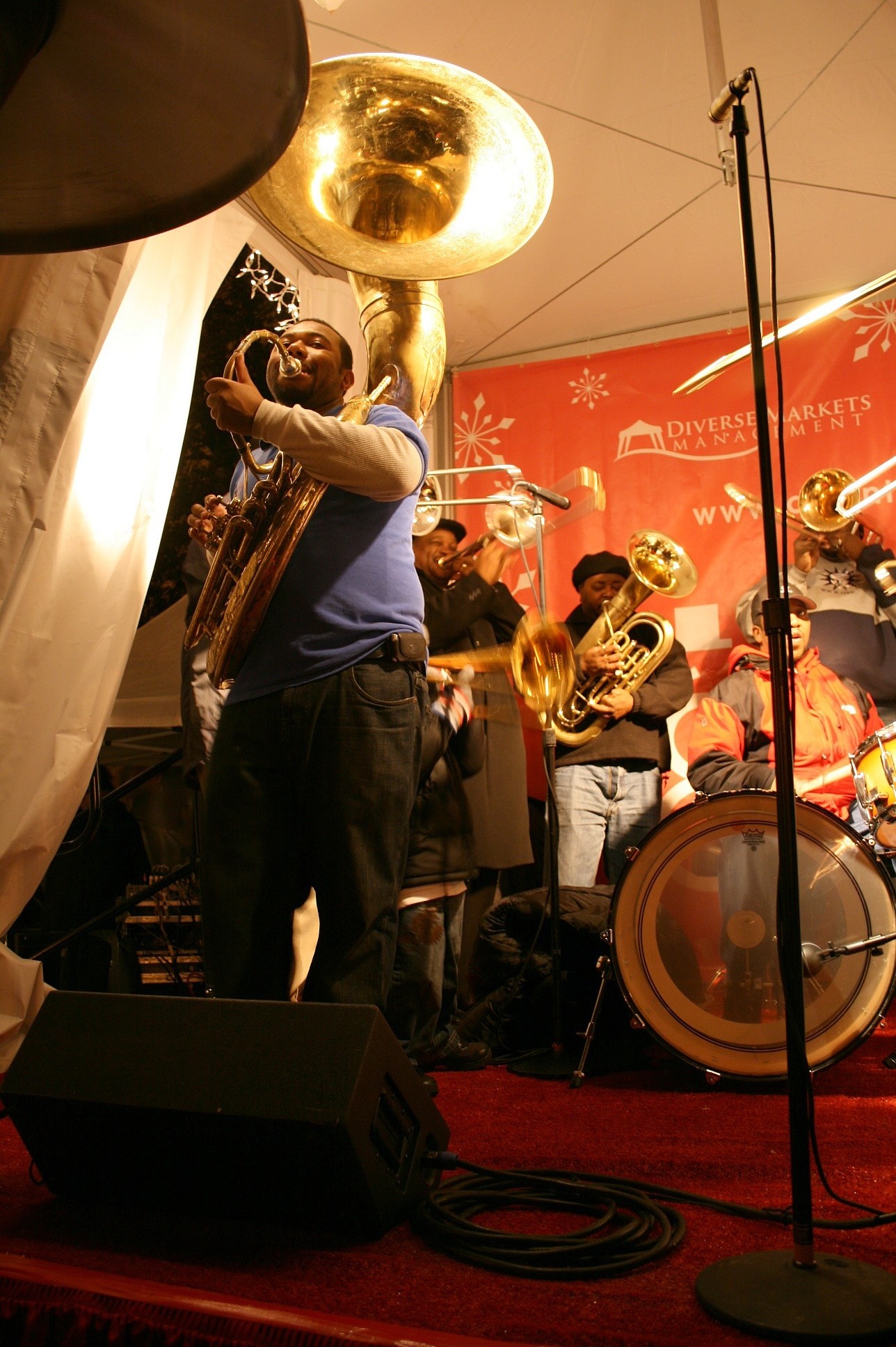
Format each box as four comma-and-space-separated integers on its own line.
572, 550, 632, 592
750, 579, 817, 617
435, 517, 467, 545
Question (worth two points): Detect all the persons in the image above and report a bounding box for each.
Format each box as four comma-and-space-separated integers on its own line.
186, 316, 432, 1014
385, 518, 534, 1069
687, 582, 883, 825
548, 551, 696, 892
734, 522, 896, 728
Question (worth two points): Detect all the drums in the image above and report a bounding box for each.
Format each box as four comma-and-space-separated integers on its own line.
602, 787, 896, 1087
846, 719, 896, 852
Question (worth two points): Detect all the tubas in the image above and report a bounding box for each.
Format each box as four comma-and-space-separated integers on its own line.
536, 529, 702, 749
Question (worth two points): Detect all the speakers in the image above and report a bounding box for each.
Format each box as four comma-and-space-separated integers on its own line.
0, 988, 446, 1255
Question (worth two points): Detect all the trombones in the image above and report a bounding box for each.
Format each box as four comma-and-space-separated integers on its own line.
836, 440, 896, 520
411, 465, 526, 537
722, 465, 862, 556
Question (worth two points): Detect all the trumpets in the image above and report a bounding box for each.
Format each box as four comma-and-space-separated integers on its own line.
435, 485, 547, 579
415, 609, 583, 723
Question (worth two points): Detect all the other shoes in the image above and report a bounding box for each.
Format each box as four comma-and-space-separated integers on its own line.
410, 1063, 439, 1094
431, 1041, 491, 1070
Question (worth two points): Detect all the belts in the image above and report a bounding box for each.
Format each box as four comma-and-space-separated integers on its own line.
366, 642, 428, 677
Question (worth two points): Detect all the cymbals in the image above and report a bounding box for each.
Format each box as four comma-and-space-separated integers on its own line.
1, 1, 310, 255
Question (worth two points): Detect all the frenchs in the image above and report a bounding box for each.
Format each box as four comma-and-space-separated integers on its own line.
179, 49, 556, 694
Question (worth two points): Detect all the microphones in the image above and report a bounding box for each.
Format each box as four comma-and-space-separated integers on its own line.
706, 67, 754, 123
524, 482, 570, 510
